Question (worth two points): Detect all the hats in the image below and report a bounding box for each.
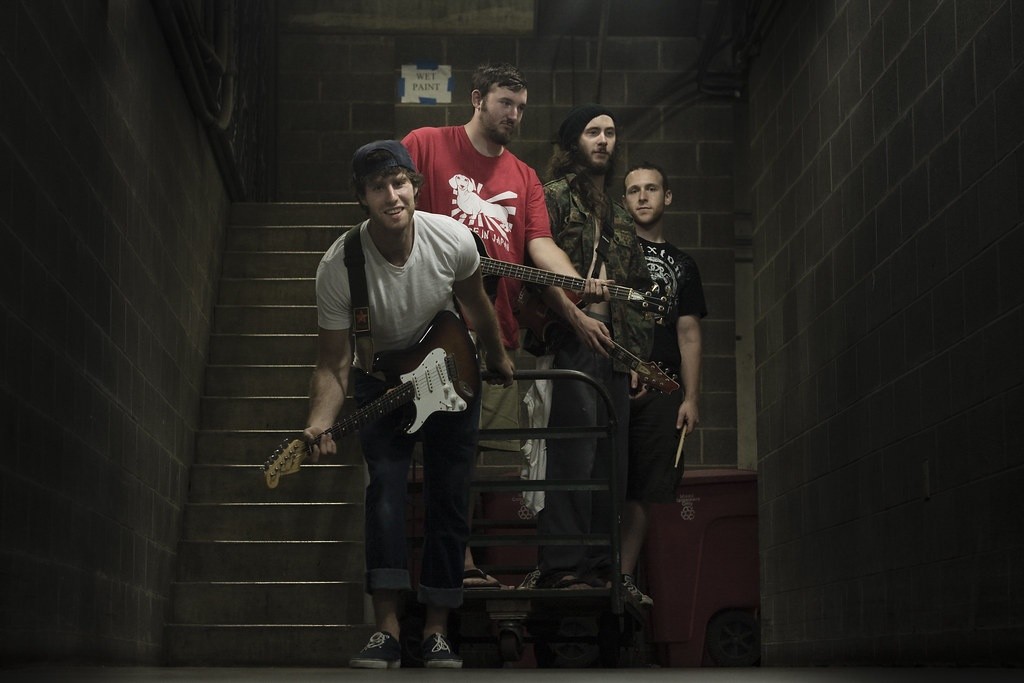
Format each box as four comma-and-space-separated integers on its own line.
348, 139, 417, 182
558, 103, 615, 150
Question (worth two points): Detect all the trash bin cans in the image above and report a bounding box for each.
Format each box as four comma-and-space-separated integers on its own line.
640, 465, 759, 666
478, 474, 539, 662
403, 467, 428, 665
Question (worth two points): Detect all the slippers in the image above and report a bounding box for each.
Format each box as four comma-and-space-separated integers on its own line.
462, 568, 505, 588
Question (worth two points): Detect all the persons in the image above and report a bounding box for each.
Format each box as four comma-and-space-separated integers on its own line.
528, 106, 652, 594
301, 140, 518, 669
613, 162, 707, 603
399, 64, 615, 586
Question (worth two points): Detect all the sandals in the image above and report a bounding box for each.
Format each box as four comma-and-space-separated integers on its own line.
541, 571, 594, 591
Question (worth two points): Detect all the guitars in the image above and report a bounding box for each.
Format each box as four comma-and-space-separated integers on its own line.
468, 228, 679, 326
514, 286, 681, 399
259, 309, 482, 488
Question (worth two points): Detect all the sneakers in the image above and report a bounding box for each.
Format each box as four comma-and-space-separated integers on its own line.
349, 631, 401, 670
606, 575, 653, 608
421, 632, 463, 669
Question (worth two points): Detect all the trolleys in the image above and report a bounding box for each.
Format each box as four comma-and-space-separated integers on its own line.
409, 368, 644, 671
